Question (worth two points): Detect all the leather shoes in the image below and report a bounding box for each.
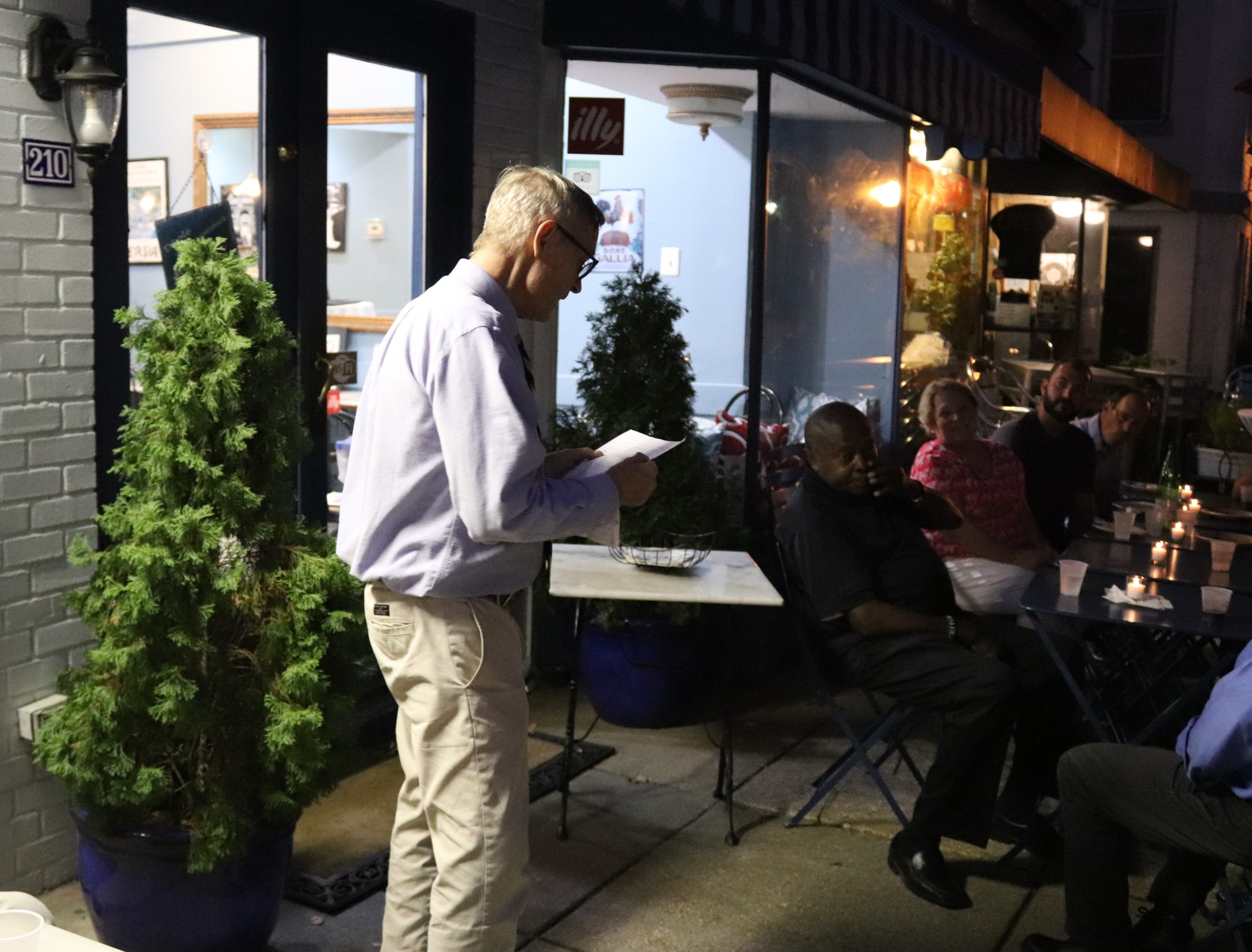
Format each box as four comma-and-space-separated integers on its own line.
988, 797, 1067, 865
887, 828, 962, 908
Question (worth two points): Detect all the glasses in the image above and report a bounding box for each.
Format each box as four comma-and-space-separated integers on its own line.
1113, 405, 1146, 434
555, 222, 599, 283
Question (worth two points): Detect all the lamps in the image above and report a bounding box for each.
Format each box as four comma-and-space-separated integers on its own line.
25, 14, 123, 166
1183, 498, 1201, 523
1170, 519, 1186, 544
657, 83, 754, 141
1150, 538, 1169, 566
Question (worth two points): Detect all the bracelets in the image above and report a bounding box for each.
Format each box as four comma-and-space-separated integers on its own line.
912, 480, 926, 503
943, 615, 956, 639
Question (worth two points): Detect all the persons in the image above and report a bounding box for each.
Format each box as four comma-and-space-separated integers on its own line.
772, 357, 1251, 952
337, 162, 659, 952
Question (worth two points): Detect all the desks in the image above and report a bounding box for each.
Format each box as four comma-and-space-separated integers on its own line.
1055, 481, 1252, 594
1022, 569, 1252, 952
1000, 358, 1137, 407
1106, 362, 1207, 434
547, 543, 785, 851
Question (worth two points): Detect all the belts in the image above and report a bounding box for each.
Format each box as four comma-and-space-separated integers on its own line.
486, 588, 524, 608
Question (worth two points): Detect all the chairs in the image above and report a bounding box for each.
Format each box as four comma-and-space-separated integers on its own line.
968, 355, 1036, 441
1225, 364, 1252, 407
773, 538, 925, 828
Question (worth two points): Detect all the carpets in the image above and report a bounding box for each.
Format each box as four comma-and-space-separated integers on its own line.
285, 729, 619, 916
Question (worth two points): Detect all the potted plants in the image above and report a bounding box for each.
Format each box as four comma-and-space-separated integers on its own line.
573, 261, 720, 731
33, 224, 364, 952
1198, 402, 1252, 480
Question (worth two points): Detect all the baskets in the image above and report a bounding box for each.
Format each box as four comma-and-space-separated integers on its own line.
606, 527, 719, 573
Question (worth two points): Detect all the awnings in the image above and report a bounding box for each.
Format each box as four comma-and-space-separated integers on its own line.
541, 2, 1042, 166
986, 64, 1189, 212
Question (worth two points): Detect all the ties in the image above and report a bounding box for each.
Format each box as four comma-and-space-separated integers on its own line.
514, 331, 543, 443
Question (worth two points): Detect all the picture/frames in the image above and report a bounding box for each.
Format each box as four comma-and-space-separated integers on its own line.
127, 156, 169, 265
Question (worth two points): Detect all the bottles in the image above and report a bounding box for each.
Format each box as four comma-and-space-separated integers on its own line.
1155, 440, 1182, 528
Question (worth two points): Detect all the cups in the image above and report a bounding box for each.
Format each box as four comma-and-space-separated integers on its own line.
1171, 521, 1187, 541
1176, 509, 1197, 537
1151, 540, 1168, 564
1188, 498, 1202, 513
1181, 484, 1194, 504
1113, 511, 1138, 541
1146, 508, 1167, 536
1201, 586, 1233, 614
1240, 485, 1252, 502
1211, 540, 1237, 572
1125, 575, 1148, 602
1059, 559, 1089, 597
0, 909, 44, 952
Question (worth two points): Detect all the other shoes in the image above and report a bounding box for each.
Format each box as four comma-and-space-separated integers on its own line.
1021, 933, 1095, 952
1131, 904, 1195, 952
1216, 888, 1252, 918
